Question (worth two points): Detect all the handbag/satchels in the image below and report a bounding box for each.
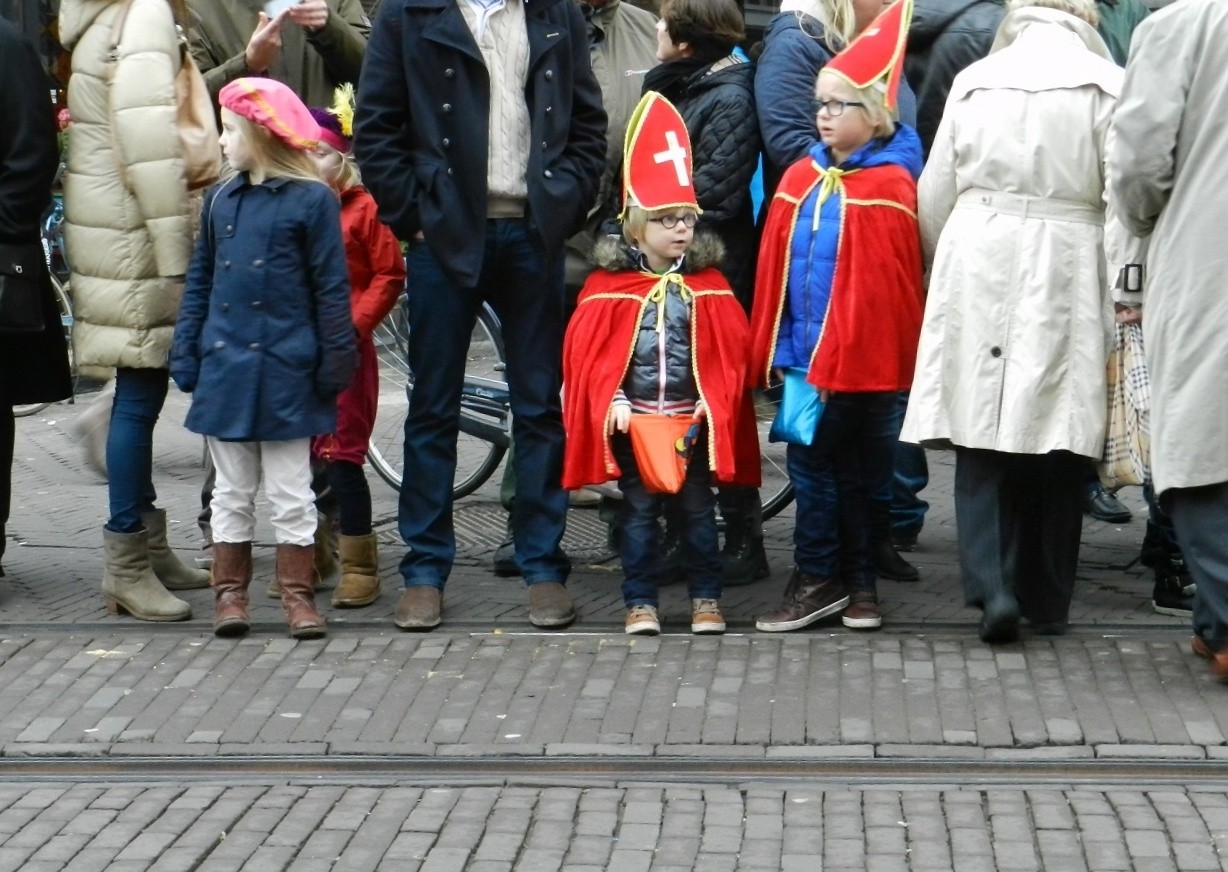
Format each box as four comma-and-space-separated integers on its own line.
628, 412, 705, 493
767, 367, 827, 444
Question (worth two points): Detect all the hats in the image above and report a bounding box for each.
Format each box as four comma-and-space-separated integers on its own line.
218, 76, 322, 152
309, 81, 357, 154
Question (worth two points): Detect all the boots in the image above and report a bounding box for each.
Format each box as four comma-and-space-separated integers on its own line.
870, 502, 920, 580
266, 516, 340, 598
657, 498, 684, 585
276, 543, 328, 638
101, 507, 211, 621
1152, 566, 1196, 617
713, 488, 772, 586
330, 528, 383, 608
211, 540, 253, 634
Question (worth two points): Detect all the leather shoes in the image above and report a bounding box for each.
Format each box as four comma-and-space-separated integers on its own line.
1086, 488, 1133, 522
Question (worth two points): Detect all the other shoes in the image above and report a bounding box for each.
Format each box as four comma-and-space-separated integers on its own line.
393, 584, 444, 629
528, 581, 576, 626
493, 511, 522, 574
1192, 634, 1228, 685
968, 585, 1068, 644
195, 535, 215, 569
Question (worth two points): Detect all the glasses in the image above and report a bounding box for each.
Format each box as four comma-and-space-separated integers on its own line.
806, 98, 864, 117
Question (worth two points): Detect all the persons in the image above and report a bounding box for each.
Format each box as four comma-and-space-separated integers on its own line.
0, 2, 1226, 687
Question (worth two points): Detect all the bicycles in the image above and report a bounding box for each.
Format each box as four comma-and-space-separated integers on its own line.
364, 252, 794, 535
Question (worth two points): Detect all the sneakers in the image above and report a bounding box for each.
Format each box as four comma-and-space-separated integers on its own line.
691, 598, 726, 633
755, 564, 882, 632
624, 604, 661, 635
